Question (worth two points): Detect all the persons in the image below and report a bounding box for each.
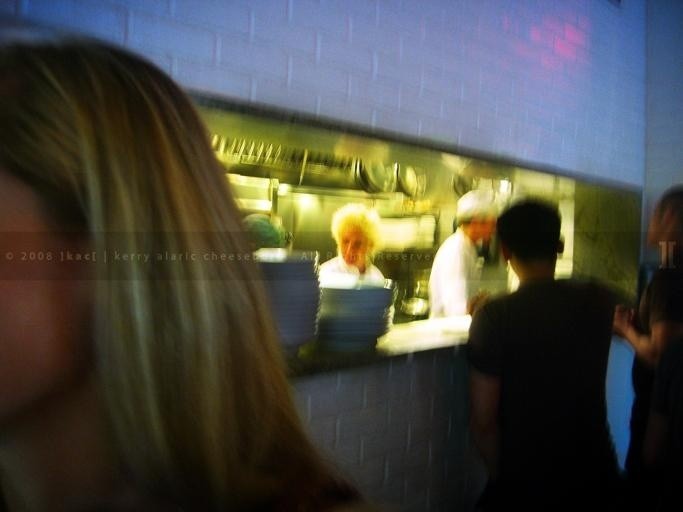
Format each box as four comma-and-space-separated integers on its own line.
467, 198, 645, 512
312, 202, 388, 284
425, 187, 500, 319
611, 181, 683, 512
0, 32, 371, 512
243, 211, 285, 252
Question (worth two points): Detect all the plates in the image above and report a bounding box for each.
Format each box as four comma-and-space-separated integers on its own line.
254, 244, 394, 361
351, 155, 426, 197
